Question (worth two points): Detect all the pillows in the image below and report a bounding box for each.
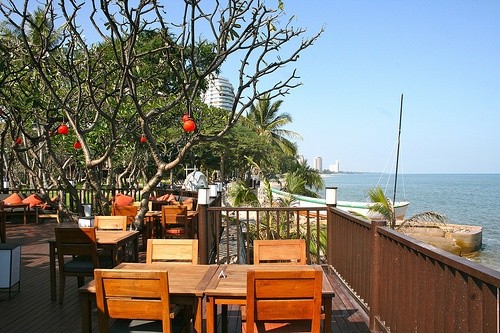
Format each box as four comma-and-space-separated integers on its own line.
2, 192, 58, 208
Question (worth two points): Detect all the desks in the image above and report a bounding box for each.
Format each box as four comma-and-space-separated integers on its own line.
203, 264, 335, 333
145, 210, 198, 245
4, 203, 31, 225
78, 263, 220, 333
47, 230, 140, 305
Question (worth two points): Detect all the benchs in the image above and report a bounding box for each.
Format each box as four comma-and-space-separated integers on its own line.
0, 193, 45, 224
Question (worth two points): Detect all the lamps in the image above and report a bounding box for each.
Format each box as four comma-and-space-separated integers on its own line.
78, 217, 95, 227
325, 187, 338, 207
197, 180, 222, 205
0, 239, 20, 301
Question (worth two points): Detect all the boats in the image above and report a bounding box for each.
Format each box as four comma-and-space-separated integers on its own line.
264, 186, 409, 220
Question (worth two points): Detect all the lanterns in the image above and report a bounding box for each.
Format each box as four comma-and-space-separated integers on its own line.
183, 114, 196, 132
17, 138, 21, 144
74, 142, 82, 149
58, 125, 68, 140
141, 137, 146, 142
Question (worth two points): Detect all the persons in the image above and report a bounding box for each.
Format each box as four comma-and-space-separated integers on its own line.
141, 190, 179, 205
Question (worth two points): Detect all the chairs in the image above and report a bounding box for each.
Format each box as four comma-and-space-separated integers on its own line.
54, 194, 323, 333
31, 189, 64, 224
